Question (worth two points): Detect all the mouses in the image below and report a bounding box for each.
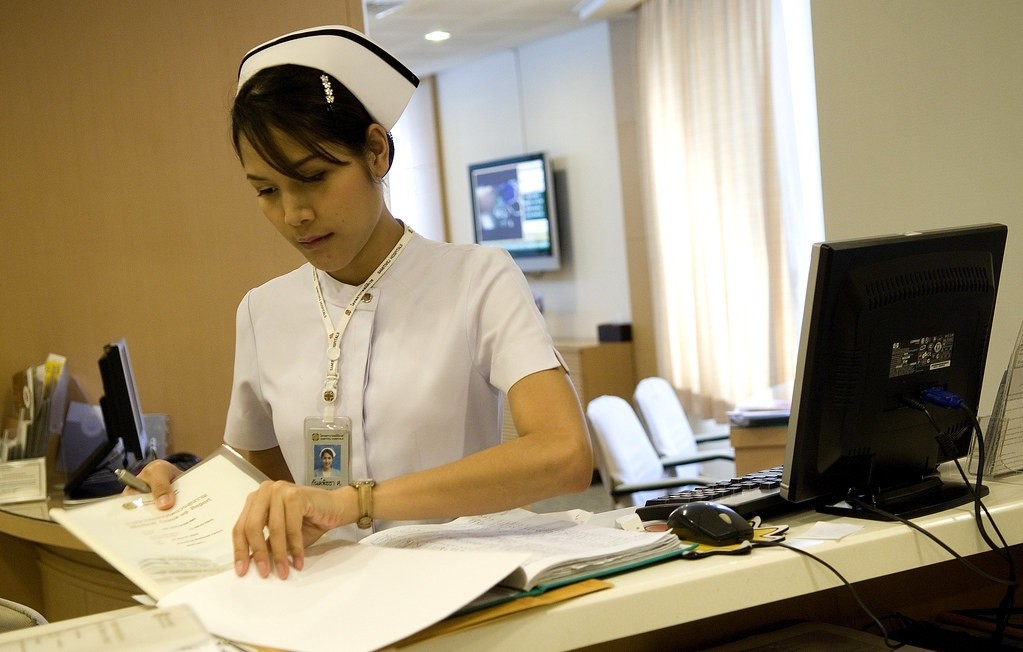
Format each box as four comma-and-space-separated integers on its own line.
166, 451, 201, 472
668, 501, 754, 547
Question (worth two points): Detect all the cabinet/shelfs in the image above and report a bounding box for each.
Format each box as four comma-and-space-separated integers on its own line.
495, 335, 635, 472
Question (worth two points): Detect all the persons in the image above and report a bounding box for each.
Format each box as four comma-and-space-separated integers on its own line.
117, 24, 592, 578
313, 447, 338, 478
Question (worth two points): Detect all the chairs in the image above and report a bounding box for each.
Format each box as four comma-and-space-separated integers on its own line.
581, 394, 740, 509
630, 375, 732, 480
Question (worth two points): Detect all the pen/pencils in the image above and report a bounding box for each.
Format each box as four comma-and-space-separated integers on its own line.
114, 468, 151, 494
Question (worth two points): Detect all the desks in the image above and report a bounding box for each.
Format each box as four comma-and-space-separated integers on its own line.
0, 468, 155, 619
385, 457, 1023, 652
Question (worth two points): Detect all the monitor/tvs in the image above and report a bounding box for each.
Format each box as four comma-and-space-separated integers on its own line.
780, 222, 1008, 523
64, 336, 150, 502
469, 152, 560, 272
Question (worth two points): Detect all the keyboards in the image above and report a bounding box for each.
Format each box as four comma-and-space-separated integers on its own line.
635, 465, 844, 522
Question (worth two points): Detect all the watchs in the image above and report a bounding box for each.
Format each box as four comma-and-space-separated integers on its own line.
347, 475, 377, 528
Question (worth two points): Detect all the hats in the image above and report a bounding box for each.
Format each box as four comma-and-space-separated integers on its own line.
233, 25, 420, 135
319, 448, 336, 457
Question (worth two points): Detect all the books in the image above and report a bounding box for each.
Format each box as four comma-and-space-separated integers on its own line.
160, 508, 698, 652
